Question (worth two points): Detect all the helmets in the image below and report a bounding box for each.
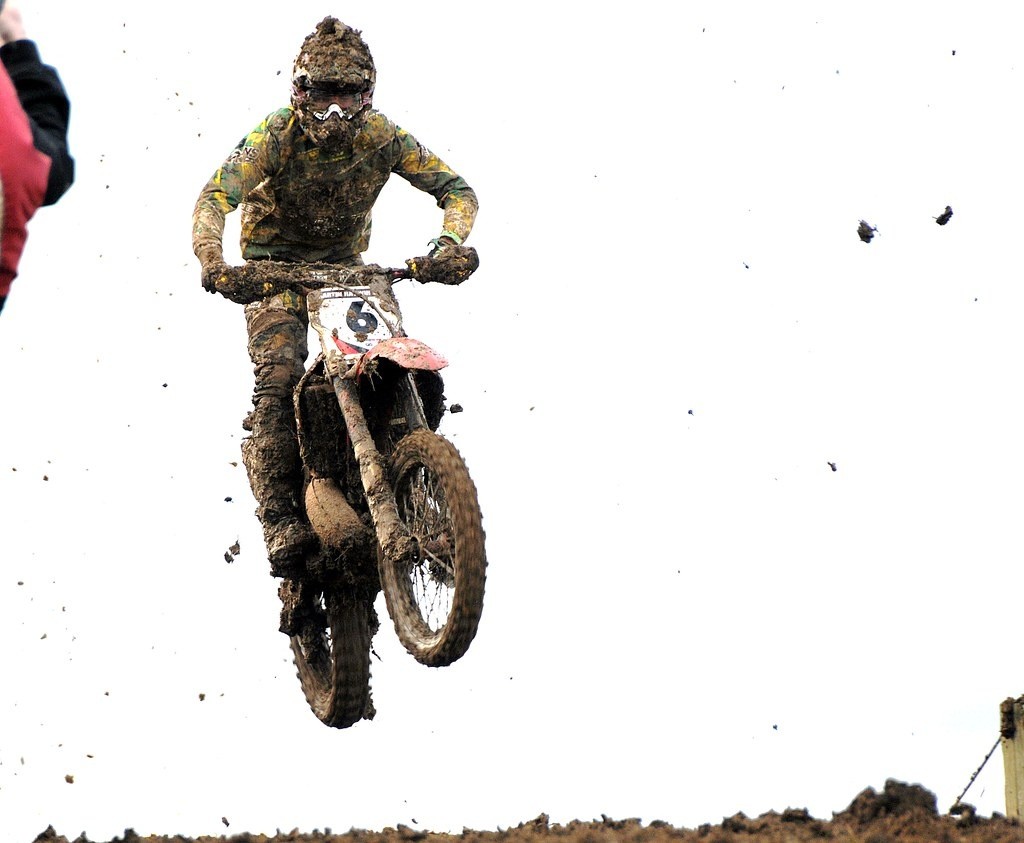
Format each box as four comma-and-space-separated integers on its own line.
291, 15, 376, 155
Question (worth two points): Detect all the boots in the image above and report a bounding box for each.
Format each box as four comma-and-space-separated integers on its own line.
240, 437, 313, 574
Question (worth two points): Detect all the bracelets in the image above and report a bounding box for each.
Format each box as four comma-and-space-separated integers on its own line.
440, 230, 461, 243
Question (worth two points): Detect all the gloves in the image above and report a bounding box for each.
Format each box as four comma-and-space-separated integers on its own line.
197, 244, 228, 294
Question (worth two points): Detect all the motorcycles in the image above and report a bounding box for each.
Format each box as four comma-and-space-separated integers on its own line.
207, 246, 488, 731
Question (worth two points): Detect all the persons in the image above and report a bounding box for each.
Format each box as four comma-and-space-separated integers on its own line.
193, 16, 479, 562
0, 0, 75, 311
